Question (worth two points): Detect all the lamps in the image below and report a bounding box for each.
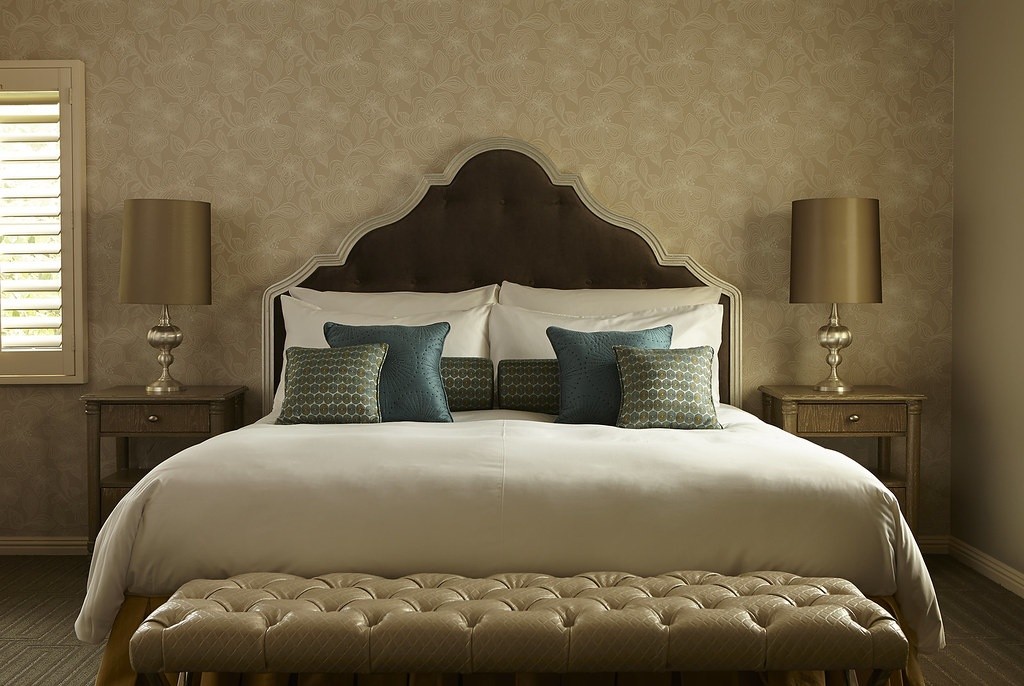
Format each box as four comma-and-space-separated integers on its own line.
790, 197, 883, 393
119, 199, 212, 394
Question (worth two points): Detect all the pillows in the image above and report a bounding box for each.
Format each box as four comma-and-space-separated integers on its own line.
546, 324, 673, 425
440, 357, 496, 412
499, 281, 723, 315
275, 343, 389, 425
288, 284, 500, 317
324, 322, 455, 423
265, 295, 496, 423
489, 302, 723, 406
498, 359, 561, 414
612, 344, 725, 430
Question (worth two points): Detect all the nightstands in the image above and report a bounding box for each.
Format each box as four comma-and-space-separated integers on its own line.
79, 384, 249, 553
758, 384, 928, 543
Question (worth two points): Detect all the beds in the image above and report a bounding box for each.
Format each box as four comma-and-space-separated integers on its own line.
75, 134, 948, 686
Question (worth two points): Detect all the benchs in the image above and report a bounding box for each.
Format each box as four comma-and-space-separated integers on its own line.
129, 568, 910, 686
